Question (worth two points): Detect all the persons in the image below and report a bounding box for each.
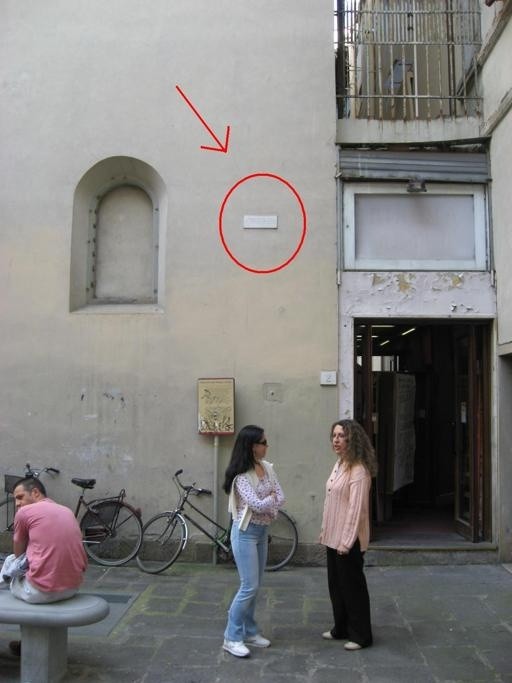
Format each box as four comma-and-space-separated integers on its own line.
222, 424, 285, 656
335, 45, 349, 120
318, 419, 379, 651
0, 477, 87, 656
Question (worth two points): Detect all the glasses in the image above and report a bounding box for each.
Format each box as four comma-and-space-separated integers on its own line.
255, 439, 268, 446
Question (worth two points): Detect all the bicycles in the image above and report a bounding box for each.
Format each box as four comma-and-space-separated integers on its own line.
0, 460, 145, 569
133, 465, 303, 576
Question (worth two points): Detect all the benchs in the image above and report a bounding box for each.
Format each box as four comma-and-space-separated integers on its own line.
0, 590, 110, 683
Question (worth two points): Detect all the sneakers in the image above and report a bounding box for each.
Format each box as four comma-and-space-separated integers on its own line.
344, 642, 362, 651
9, 640, 22, 655
245, 633, 271, 649
222, 636, 251, 658
322, 630, 335, 639
